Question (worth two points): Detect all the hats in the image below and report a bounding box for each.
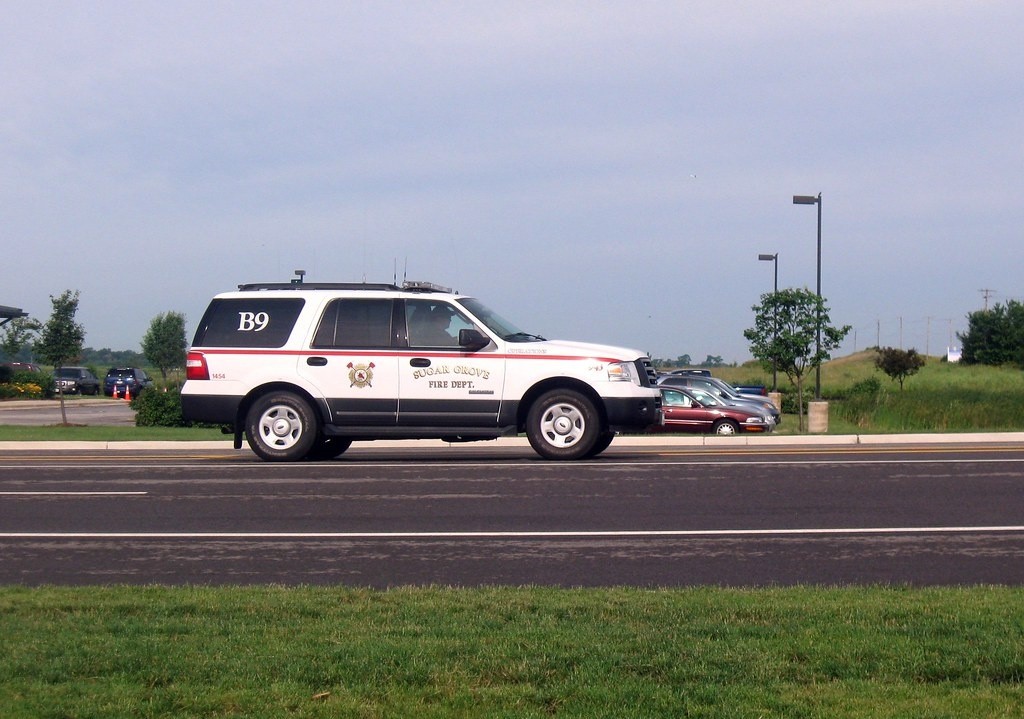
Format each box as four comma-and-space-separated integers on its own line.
430, 305, 456, 317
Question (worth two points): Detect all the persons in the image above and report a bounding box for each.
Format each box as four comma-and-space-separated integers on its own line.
430, 304, 458, 346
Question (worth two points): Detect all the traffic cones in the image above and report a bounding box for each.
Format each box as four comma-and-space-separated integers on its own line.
123, 384, 132, 400
112, 384, 119, 399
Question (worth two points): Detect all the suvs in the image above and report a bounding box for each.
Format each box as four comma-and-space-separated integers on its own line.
47, 366, 100, 398
104, 366, 156, 399
180, 266, 665, 462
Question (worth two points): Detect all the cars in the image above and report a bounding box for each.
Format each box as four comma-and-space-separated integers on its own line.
651, 365, 783, 437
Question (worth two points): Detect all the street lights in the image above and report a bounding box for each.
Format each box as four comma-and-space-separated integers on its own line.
758, 252, 778, 393
793, 191, 822, 401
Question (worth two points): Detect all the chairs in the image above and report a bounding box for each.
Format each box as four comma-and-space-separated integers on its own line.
409, 306, 432, 346
